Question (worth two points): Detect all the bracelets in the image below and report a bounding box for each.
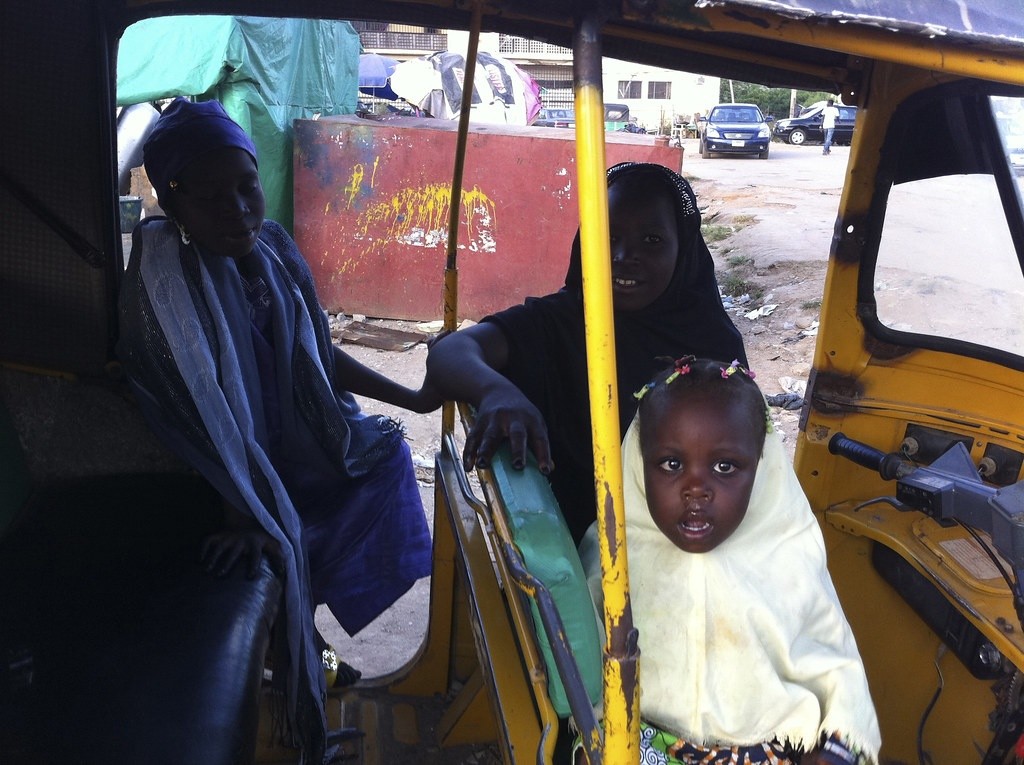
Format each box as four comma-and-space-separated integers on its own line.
819, 126, 822, 129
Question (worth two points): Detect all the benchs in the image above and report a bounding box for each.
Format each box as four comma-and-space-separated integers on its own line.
458, 392, 604, 737
0, 437, 282, 765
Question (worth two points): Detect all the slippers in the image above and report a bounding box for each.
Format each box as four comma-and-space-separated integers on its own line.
262, 649, 357, 693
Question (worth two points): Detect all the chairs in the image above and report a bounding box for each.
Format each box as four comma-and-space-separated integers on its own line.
742, 113, 750, 121
716, 112, 725, 120
727, 114, 737, 121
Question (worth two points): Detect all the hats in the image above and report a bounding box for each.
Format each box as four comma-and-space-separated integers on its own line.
143, 97, 257, 210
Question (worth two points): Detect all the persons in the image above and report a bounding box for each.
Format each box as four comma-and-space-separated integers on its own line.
819, 100, 841, 155
117, 98, 454, 765
428, 162, 749, 543
581, 356, 883, 765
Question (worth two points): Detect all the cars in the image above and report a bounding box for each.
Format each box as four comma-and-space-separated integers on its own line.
363, 102, 411, 116
998, 113, 1024, 177
772, 105, 858, 146
602, 102, 647, 134
532, 108, 576, 128
697, 104, 772, 159
